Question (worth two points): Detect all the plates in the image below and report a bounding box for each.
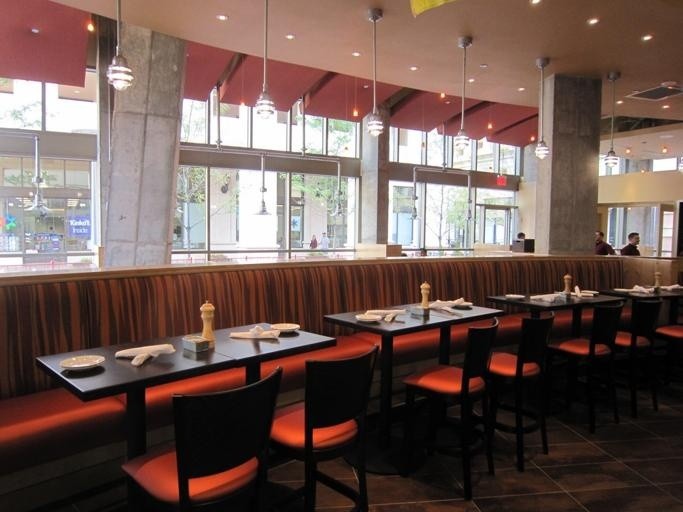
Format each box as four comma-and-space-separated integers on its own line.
612, 284, 683, 292
270, 323, 299, 333
355, 313, 381, 324
60, 355, 104, 370
504, 294, 526, 301
450, 301, 472, 309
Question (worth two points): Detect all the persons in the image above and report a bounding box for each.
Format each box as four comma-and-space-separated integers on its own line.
309, 233, 317, 250
516, 232, 527, 241
595, 230, 618, 256
318, 232, 330, 250
621, 233, 641, 256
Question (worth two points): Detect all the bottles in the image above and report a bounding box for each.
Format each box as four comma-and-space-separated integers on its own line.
419, 281, 430, 308
198, 303, 215, 341
563, 274, 573, 295
654, 272, 661, 287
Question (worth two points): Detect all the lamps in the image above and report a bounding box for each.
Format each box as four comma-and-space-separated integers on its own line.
24, 135, 52, 215
